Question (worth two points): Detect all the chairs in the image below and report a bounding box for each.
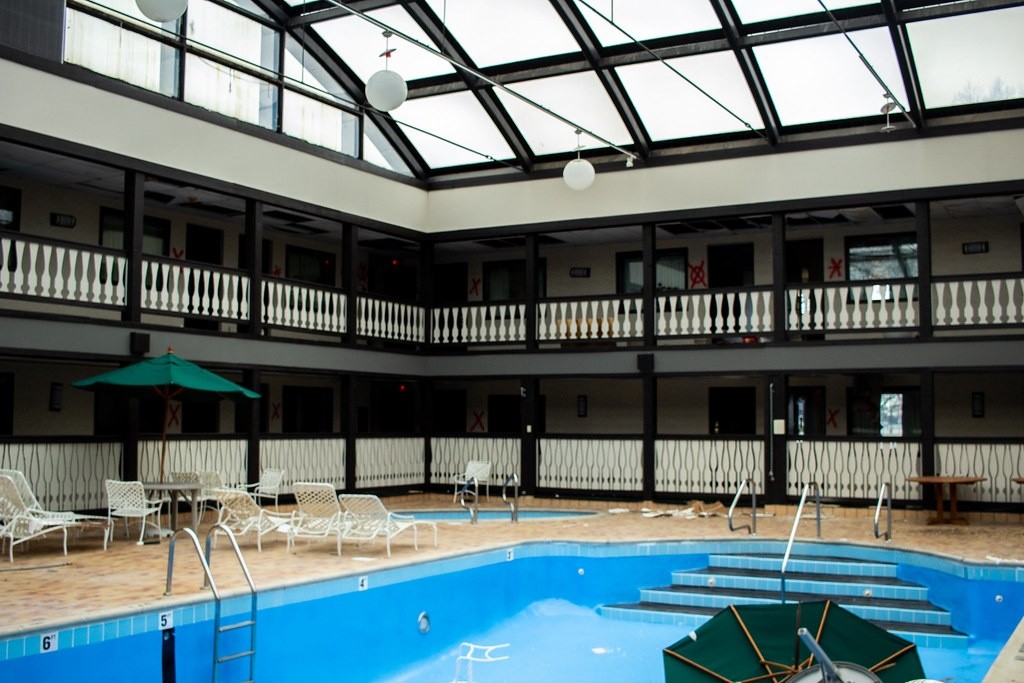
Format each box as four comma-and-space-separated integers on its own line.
0, 469, 438, 563
453, 459, 492, 505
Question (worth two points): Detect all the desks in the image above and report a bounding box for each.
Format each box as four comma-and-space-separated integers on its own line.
907, 476, 987, 526
141, 482, 204, 530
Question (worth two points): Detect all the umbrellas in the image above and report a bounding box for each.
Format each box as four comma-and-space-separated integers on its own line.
663, 596, 926, 683
70, 347, 263, 500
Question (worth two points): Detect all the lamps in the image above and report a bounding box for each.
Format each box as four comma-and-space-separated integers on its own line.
135, 0, 189, 23
563, 131, 596, 189
365, 30, 408, 109
